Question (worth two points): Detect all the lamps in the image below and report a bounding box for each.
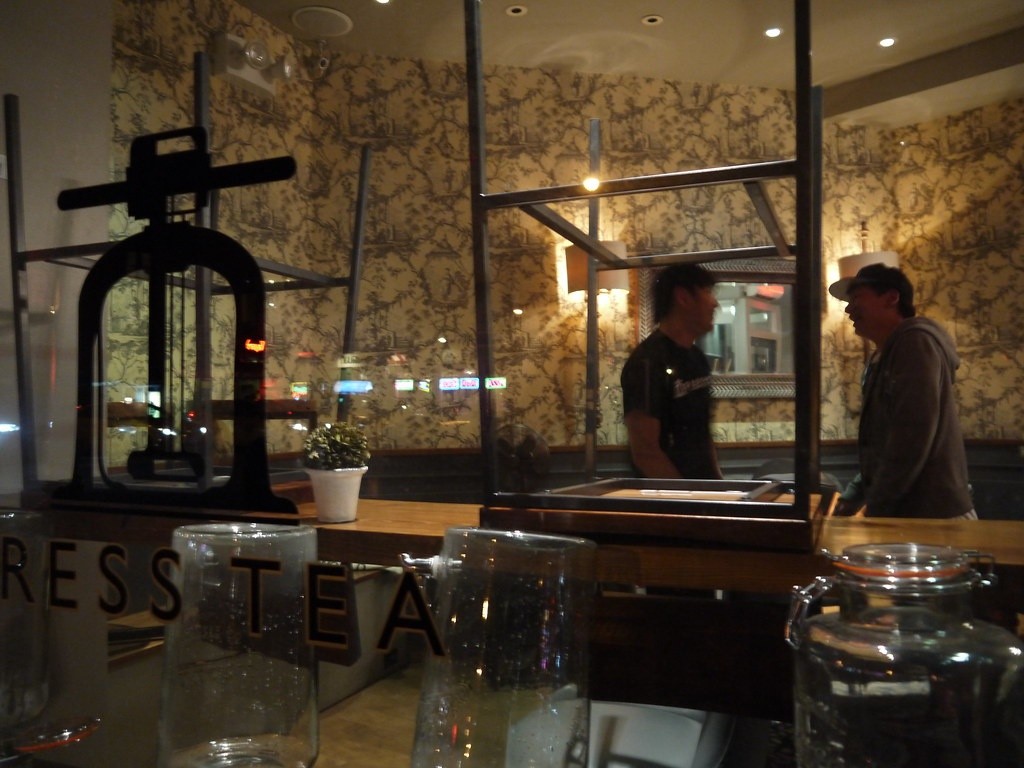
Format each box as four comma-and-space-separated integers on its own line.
212, 31, 292, 99
314, 39, 328, 69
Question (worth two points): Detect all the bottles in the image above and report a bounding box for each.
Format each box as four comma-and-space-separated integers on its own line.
783, 541, 1023, 767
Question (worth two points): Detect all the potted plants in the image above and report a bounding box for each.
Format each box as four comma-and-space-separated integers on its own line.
298, 422, 369, 523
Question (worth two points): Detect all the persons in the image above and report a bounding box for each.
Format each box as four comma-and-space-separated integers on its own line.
620, 263, 726, 480
829, 264, 980, 522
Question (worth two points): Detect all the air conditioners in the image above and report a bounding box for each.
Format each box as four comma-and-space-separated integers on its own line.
746, 283, 784, 301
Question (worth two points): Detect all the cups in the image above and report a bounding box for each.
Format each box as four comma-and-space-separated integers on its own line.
0, 507, 59, 736
161, 521, 320, 768
410, 528, 598, 767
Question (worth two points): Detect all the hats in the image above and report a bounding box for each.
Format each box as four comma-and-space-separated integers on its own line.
829, 262, 914, 301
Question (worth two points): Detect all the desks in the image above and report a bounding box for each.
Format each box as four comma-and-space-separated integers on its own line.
293, 500, 1024, 566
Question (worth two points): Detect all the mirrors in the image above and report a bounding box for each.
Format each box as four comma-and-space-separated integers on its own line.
637, 249, 795, 399
